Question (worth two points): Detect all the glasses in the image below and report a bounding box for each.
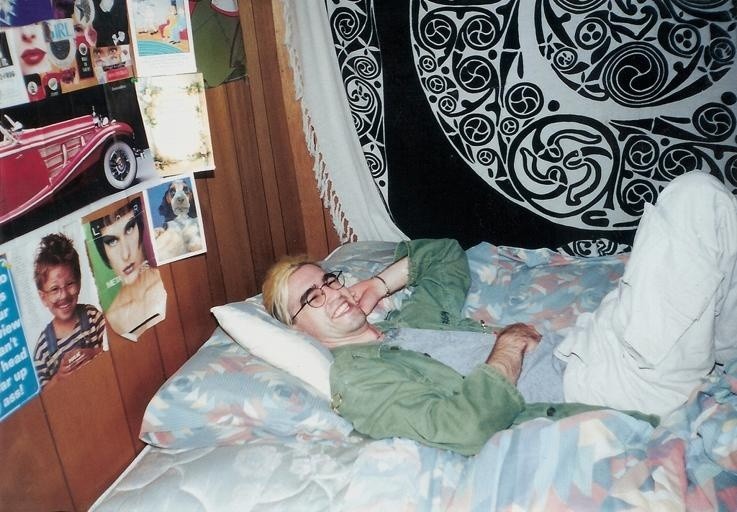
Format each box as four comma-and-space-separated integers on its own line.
36, 281, 79, 304
290, 270, 345, 322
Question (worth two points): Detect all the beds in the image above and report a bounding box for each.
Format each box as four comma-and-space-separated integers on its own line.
87, 240, 737, 512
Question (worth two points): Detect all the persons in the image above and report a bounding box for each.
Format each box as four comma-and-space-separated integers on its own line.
34, 233, 106, 387
90, 197, 167, 337
0, 26, 52, 77
262, 169, 737, 457
73, 1, 121, 68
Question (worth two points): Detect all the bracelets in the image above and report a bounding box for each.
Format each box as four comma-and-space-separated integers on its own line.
370, 276, 391, 297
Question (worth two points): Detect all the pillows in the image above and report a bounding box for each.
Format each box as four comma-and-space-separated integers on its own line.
209, 242, 411, 399
138, 323, 354, 450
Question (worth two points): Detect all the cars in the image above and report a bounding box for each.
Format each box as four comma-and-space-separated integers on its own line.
1, 111, 139, 223
